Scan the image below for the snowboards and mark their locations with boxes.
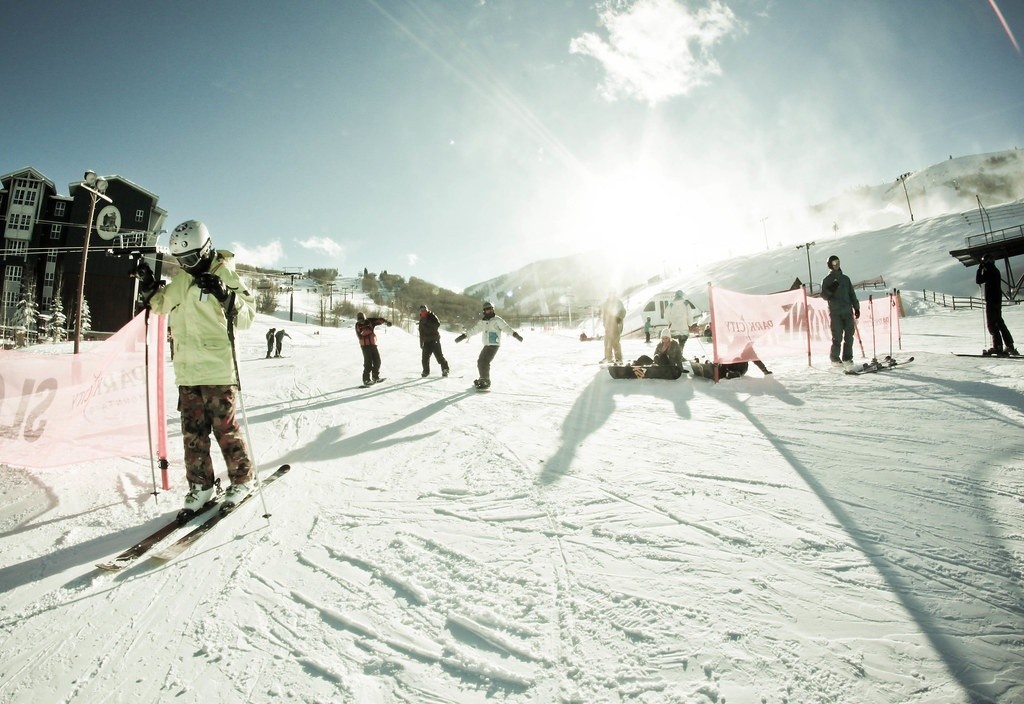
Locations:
[274,356,292,359]
[404,375,463,379]
[358,377,387,389]
[474,376,492,393]
[259,355,276,359]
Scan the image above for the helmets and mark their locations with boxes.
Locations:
[982,254,995,267]
[483,302,494,310]
[827,256,838,268]
[168,220,212,259]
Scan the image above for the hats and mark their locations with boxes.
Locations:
[661,329,670,337]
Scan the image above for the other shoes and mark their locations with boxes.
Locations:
[184,483,214,512]
[373,377,382,382]
[442,369,449,376]
[365,380,373,385]
[223,480,253,503]
[474,380,489,389]
[989,347,1003,353]
[422,373,428,377]
[1004,346,1013,353]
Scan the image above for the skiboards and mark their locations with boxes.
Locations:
[96,463,290,581]
[842,357,915,375]
[950,351,1024,358]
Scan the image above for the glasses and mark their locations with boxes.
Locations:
[832,260,839,266]
[176,253,200,268]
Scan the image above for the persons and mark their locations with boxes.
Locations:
[976,254,1014,354]
[820,256,860,366]
[598,290,772,379]
[266,328,291,358]
[418,305,450,377]
[130,219,256,522]
[355,312,393,385]
[454,301,523,389]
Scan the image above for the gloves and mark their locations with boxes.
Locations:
[831,281,840,290]
[455,334,465,343]
[196,272,228,302]
[141,270,157,293]
[512,332,523,342]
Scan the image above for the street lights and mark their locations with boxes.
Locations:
[895,171,920,224]
[72,169,113,353]
[796,240,819,295]
